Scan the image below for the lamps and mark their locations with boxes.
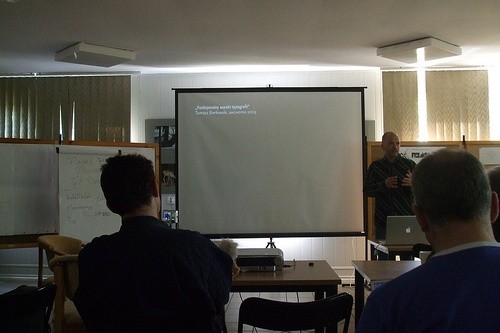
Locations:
[374,37,464,67]
[53,42,135,69]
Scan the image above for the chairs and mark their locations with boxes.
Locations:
[238,292,353,333]
[35,234,85,333]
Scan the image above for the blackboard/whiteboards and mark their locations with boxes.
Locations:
[0,137,60,249]
[59,140,160,244]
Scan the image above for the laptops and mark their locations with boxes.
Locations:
[385,215,427,246]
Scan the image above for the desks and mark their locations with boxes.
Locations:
[351,259,424,333]
[226,259,342,333]
[365,237,431,291]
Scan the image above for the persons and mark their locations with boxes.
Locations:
[363,131,417,241]
[73,152,231,333]
[359,147,499,333]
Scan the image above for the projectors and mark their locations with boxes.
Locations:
[236,248,285,272]
